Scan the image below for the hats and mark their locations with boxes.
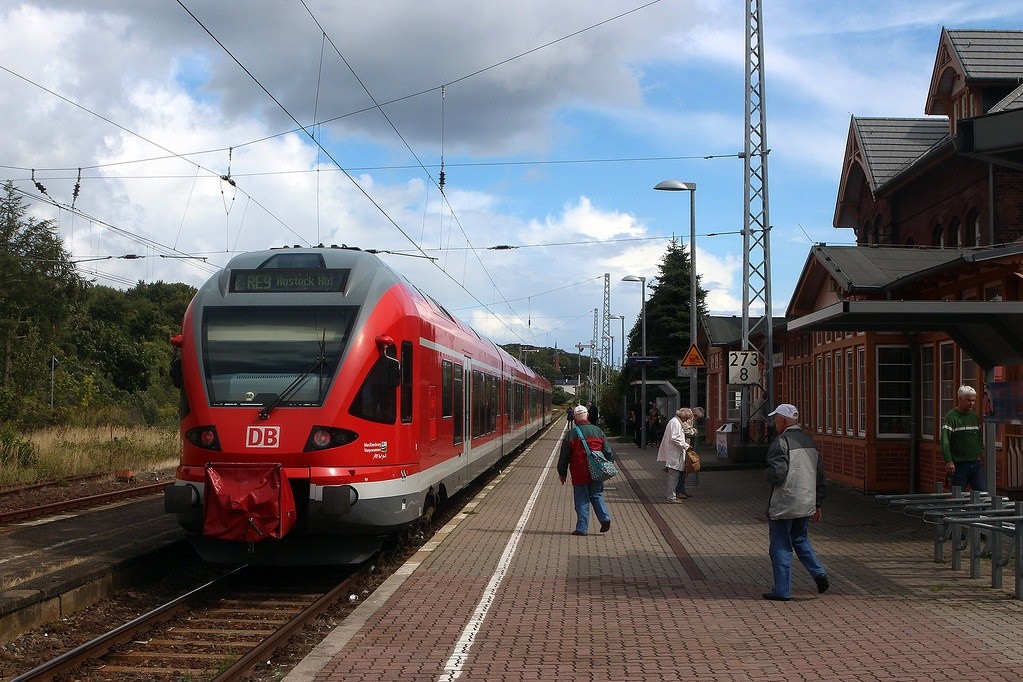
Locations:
[574,405,588,415]
[768,403,798,419]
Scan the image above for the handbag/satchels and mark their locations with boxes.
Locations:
[587,450,618,482]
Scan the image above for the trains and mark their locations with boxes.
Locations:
[163,242,554,559]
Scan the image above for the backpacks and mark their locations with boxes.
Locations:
[685,449,700,473]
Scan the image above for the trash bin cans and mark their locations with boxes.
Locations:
[716,423,740,460]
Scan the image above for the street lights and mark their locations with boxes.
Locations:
[621,275,647,448]
[653,179,699,486]
[595,313,628,439]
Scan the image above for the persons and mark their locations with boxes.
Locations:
[940,385,987,540]
[658,406,705,504]
[567,404,574,430]
[588,403,599,426]
[557,405,614,535]
[631,398,661,447]
[761,403,831,601]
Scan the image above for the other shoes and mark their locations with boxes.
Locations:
[676,491,688,499]
[668,496,683,504]
[761,591,790,601]
[682,490,692,497]
[817,573,829,594]
[600,520,610,532]
[980,532,988,541]
[572,530,587,536]
[949,531,966,541]
